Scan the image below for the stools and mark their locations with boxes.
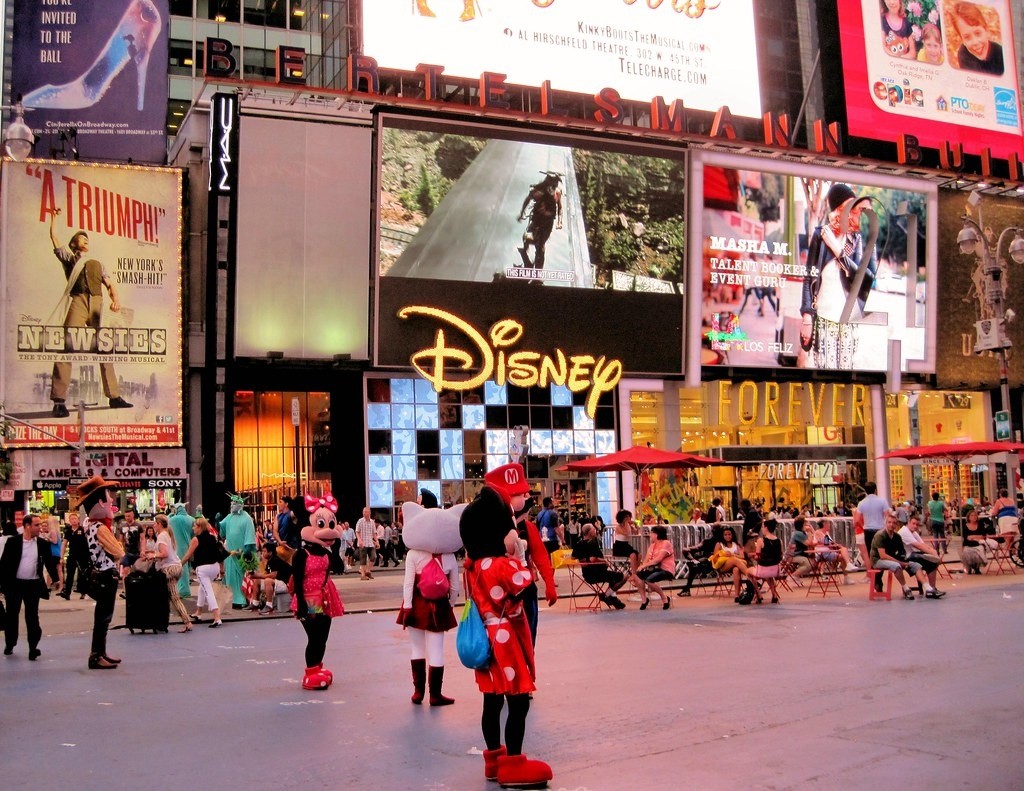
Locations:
[275,592,292,612]
[697,560,802,601]
[584,555,674,611]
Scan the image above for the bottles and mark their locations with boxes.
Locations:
[824,535,829,545]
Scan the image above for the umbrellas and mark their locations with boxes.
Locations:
[555,445,725,524]
[876,441,1024,536]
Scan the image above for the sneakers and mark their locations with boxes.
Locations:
[677,587,691,597]
[901,586,946,600]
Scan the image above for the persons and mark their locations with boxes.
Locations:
[881,0,1004,76]
[852,482,949,600]
[528,497,676,611]
[113,491,299,633]
[795,184,876,370]
[677,517,855,605]
[641,494,852,526]
[517,170,563,269]
[961,509,989,575]
[286,496,343,690]
[737,185,780,317]
[0,511,91,662]
[49,208,133,419]
[945,489,1024,552]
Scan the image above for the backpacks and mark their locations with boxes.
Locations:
[78,563,106,603]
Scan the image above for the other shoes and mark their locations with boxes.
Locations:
[47,581,85,600]
[843,575,870,585]
[119,591,126,599]
[640,596,670,610]
[242,602,275,613]
[734,595,778,604]
[177,613,223,633]
[968,568,982,574]
[786,574,804,584]
[347,560,402,580]
[4,644,41,661]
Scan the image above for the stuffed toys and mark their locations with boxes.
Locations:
[459,463,558,786]
[885,30,909,57]
[393,488,470,707]
[70,475,125,670]
[329,502,465,581]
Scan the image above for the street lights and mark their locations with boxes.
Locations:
[957,214,1024,441]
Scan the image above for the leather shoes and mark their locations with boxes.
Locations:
[89,654,121,669]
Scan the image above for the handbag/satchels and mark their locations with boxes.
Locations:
[0,600,7,631]
[217,541,231,563]
[550,544,580,569]
[133,557,152,572]
[922,552,942,564]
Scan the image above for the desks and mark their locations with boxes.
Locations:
[566,562,607,612]
[985,533,1018,575]
[923,538,953,581]
[805,549,844,597]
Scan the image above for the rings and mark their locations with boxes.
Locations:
[803,337,807,341]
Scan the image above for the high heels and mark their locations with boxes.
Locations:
[599,592,626,609]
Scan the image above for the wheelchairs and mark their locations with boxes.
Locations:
[1010,535,1024,567]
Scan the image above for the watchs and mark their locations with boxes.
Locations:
[153,554,157,559]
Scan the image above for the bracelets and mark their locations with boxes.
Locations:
[60,556,64,559]
[802,321,813,325]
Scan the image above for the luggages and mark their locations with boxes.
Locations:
[124,570,170,634]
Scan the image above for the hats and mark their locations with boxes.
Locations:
[75,475,120,508]
